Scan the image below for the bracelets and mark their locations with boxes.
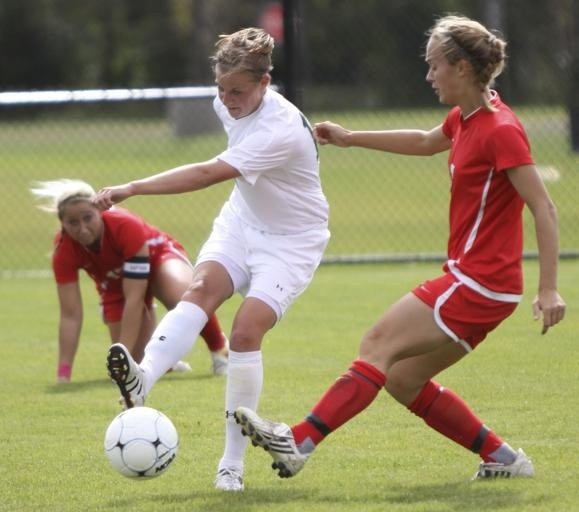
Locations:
[57,361,73,380]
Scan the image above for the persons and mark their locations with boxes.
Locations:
[90,25,327,492]
[232,11,568,485]
[29,176,230,388]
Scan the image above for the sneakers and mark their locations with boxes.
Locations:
[212,464,247,498]
[210,331,232,380]
[171,358,196,374]
[233,404,315,481]
[105,341,148,413]
[467,445,537,484]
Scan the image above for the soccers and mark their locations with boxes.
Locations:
[105,408,179,481]
